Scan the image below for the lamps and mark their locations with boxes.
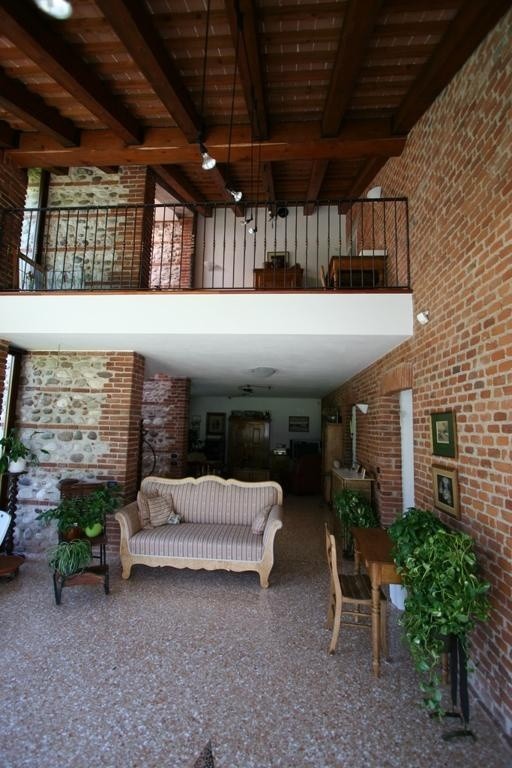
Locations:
[197,27,263,235]
[366,185,382,202]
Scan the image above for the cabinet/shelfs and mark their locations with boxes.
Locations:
[52,479,110,605]
[252,268,304,288]
[321,421,344,473]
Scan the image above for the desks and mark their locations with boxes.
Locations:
[350,526,408,678]
[328,466,376,511]
[325,255,388,290]
[1,471,27,579]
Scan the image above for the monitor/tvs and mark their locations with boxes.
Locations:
[292,440,321,460]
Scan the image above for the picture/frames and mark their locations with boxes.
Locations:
[206,412,226,436]
[428,409,463,522]
[267,250,289,265]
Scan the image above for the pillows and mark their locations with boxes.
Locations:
[137,488,174,529]
[250,504,273,536]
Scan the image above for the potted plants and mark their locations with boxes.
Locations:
[0,426,51,474]
[35,482,122,578]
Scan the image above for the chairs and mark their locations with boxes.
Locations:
[323,521,393,664]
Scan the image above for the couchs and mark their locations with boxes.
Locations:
[114,475,285,589]
